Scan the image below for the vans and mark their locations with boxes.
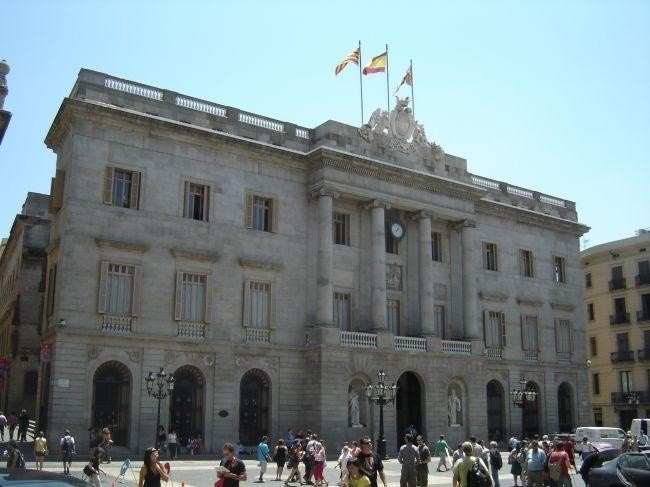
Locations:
[575,425,641,457]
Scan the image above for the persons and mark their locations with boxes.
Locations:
[434,429,502,487]
[508,430,649,486]
[393,65,412,94]
[1,410,111,486]
[363,51,387,75]
[139,425,247,487]
[335,47,360,75]
[258,428,387,486]
[398,422,431,486]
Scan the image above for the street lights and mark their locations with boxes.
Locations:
[361,368,400,460]
[509,375,538,448]
[628,392,639,425]
[144,366,177,460]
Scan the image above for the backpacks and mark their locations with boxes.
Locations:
[516,453,525,463]
[16,451,27,473]
[467,460,492,487]
[492,450,502,470]
[63,436,74,452]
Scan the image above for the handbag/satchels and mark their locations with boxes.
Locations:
[83,465,92,476]
[265,455,272,462]
[44,450,49,457]
[215,475,225,487]
[508,454,515,464]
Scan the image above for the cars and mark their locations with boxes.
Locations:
[588,450,650,487]
[552,432,576,451]
[0,471,96,486]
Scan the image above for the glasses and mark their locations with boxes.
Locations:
[362,441,373,444]
[151,449,157,453]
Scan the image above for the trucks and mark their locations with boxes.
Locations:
[631,417,650,445]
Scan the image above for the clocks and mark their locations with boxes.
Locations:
[391,222,404,241]
